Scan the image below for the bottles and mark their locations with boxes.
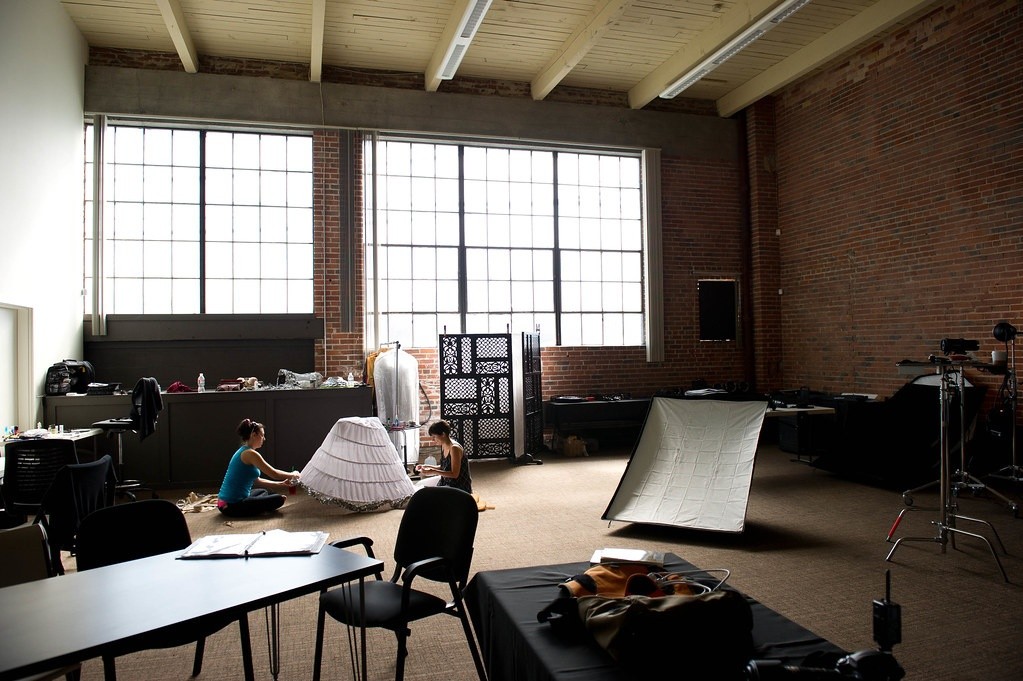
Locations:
[198,373,205,393]
[347,372,354,387]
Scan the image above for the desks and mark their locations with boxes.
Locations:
[464,548,887,681]
[763,400,835,464]
[0,526,383,681]
[1,426,105,466]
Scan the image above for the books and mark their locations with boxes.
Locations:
[600,547,666,567]
[589,549,604,566]
[175,529,331,560]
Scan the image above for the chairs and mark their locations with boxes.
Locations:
[2,437,84,518]
[0,521,81,681]
[35,456,116,553]
[308,485,487,681]
[94,378,162,493]
[73,502,255,681]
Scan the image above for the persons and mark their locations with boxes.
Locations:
[414,420,496,512]
[217,418,301,518]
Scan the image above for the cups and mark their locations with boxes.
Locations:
[991,350,1008,366]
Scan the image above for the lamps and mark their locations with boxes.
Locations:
[991,321,1023,482]
